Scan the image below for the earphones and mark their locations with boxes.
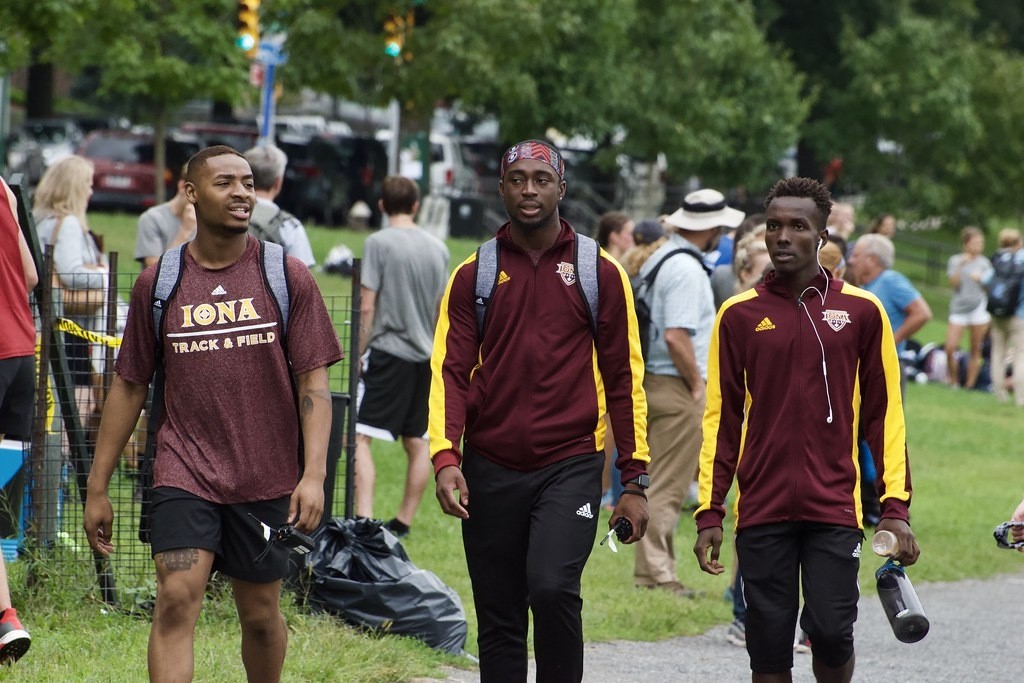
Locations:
[826,409,833,423]
[818,239,823,250]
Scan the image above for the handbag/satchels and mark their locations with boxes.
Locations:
[633,248,713,363]
[49,216,105,314]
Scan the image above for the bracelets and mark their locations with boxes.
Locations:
[619,489,647,501]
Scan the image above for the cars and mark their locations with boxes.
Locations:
[5,105,667,233]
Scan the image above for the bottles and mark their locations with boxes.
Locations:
[875,556,930,643]
[870,529,900,554]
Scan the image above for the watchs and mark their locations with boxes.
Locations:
[625,474,649,488]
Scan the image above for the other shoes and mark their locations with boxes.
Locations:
[653,582,705,600]
[728,620,747,648]
[796,631,813,657]
[124,463,140,480]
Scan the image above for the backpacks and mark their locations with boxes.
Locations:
[987,249,1024,318]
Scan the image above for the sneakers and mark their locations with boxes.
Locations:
[0,607,32,666]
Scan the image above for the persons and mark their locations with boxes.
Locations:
[634,190,744,596]
[431,138,650,683]
[592,187,1024,549]
[349,176,448,540]
[691,177,920,683]
[33,144,315,502]
[82,144,345,683]
[0,176,38,666]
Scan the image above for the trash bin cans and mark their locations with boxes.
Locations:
[319,386,350,524]
[444,191,483,240]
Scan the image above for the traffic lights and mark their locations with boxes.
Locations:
[383,13,403,58]
[234,0,257,52]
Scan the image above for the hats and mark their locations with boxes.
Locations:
[817,243,846,281]
[666,189,746,232]
[633,220,671,246]
